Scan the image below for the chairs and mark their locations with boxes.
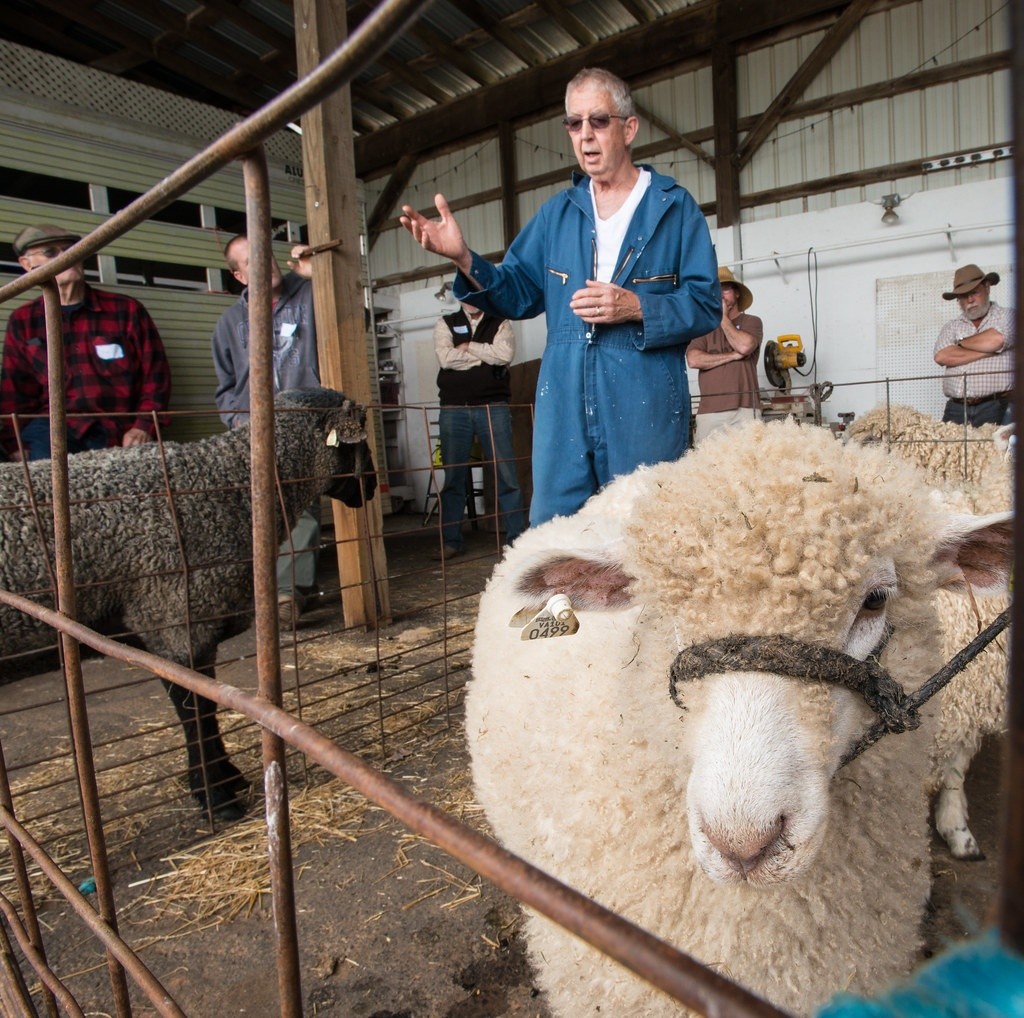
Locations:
[423,421,485,531]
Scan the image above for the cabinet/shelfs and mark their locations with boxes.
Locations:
[372,292,414,503]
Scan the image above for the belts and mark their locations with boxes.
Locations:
[952,391,1015,406]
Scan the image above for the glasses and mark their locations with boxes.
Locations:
[27,245,71,258]
[722,286,736,295]
[561,110,627,132]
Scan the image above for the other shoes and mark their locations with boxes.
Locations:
[433,546,459,562]
[279,597,299,632]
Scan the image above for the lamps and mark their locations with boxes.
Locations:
[880,194,899,225]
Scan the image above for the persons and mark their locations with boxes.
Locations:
[435,301,529,561]
[213,235,323,632]
[400,68,724,532]
[687,266,764,447]
[0,225,172,462]
[933,264,1015,427]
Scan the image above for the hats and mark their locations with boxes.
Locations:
[14,223,81,256]
[943,264,1000,300]
[716,265,753,312]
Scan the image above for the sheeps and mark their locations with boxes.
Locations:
[464,419,1013,1018]
[839,403,1015,520]
[0,387,379,824]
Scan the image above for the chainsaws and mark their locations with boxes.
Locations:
[763,334,807,393]
